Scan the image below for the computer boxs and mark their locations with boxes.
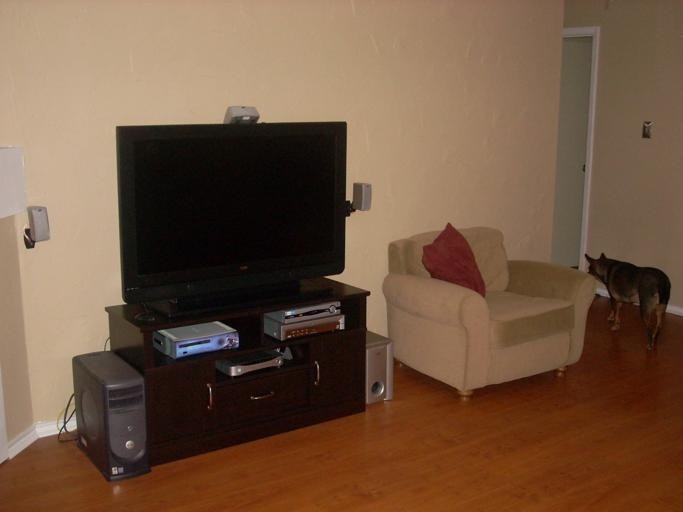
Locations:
[71,351,151,481]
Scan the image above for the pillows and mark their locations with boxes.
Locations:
[421,220,487,300]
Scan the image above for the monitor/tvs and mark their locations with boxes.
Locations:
[115,120,348,318]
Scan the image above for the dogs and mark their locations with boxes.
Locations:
[584,251,671,350]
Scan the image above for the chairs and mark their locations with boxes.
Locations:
[383,225,600,398]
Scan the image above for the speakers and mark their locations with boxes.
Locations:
[26,206,50,243]
[352,182,371,211]
[365,330,394,403]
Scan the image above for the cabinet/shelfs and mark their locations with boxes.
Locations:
[104,277,370,468]
